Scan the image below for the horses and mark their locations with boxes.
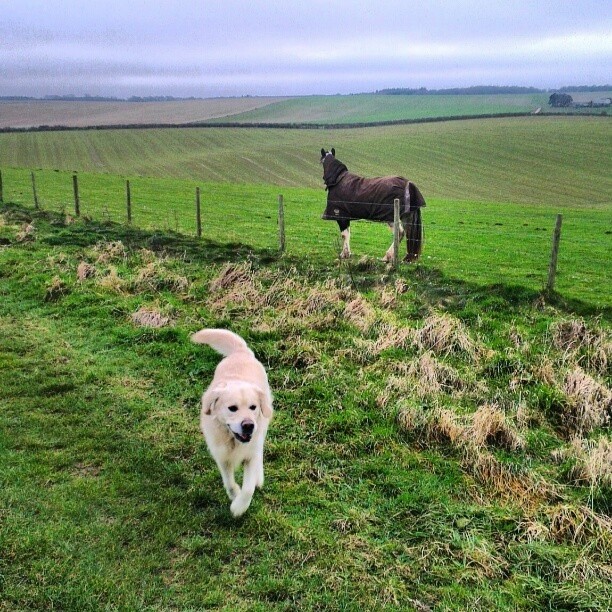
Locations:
[320,148,426,262]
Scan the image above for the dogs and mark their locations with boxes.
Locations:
[190,328,275,517]
